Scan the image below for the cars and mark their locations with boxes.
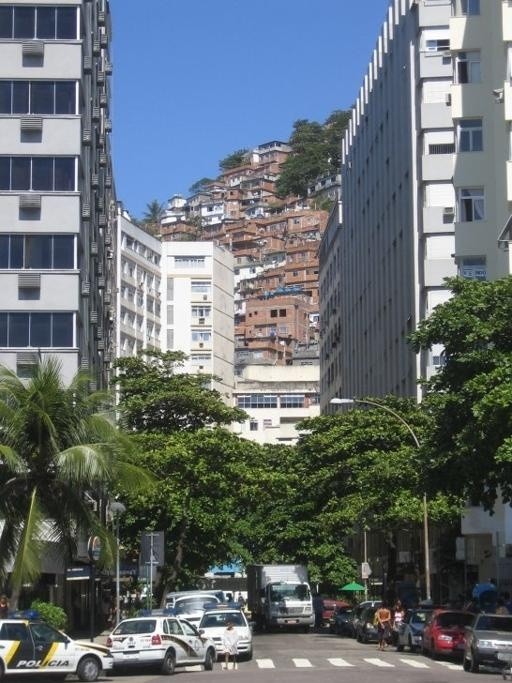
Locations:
[317,598,511,682]
[106,587,255,673]
[0,610,112,683]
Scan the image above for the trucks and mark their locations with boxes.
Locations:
[245,562,317,633]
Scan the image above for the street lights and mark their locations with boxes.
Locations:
[327,397,435,606]
[109,502,126,625]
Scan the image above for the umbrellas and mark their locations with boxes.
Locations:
[339,579,368,591]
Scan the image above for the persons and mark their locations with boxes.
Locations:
[0,595,10,619]
[373,596,406,652]
[467,595,481,614]
[220,618,240,670]
[495,599,511,616]
[314,592,325,632]
